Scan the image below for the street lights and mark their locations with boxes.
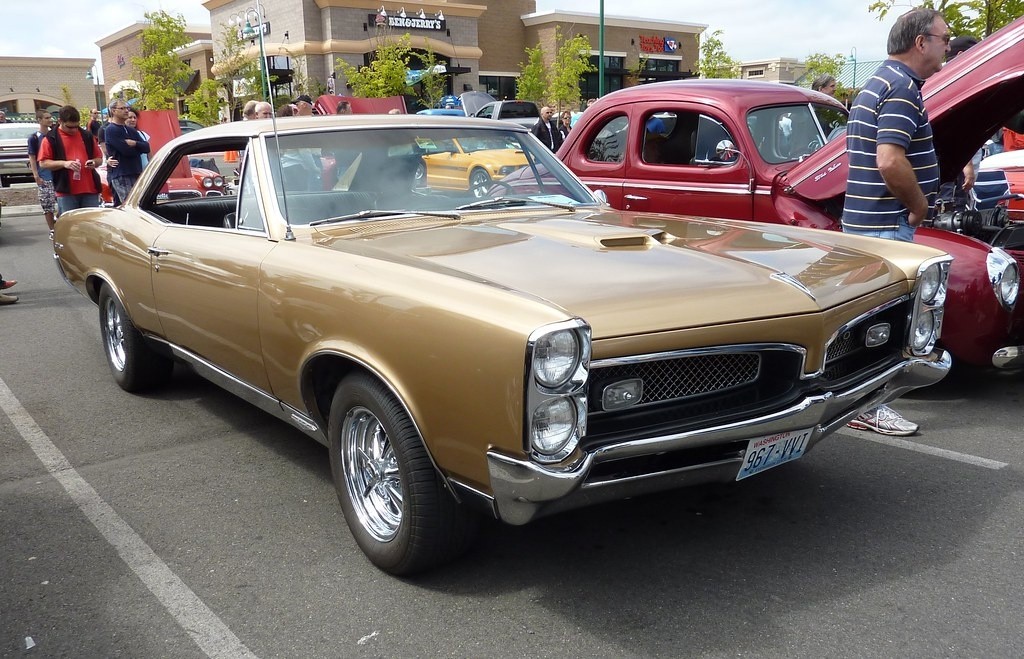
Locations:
[847,46,856,103]
[243,10,267,102]
[86,64,101,111]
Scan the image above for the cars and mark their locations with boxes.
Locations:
[415,108,466,116]
[313,94,410,190]
[415,136,536,199]
[0,121,41,188]
[48,115,952,578]
[96,108,226,207]
[178,120,239,183]
[488,17,1024,392]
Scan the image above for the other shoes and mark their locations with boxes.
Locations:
[1,279,18,290]
[49,229,55,240]
[0,293,19,304]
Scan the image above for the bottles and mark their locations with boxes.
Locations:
[72,159,81,180]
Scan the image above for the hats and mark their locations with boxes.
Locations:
[290,95,312,105]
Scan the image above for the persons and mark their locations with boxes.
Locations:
[930,34,1024,212]
[533,107,560,163]
[842,6,951,437]
[558,110,572,149]
[747,76,836,164]
[645,115,665,133]
[237,94,353,191]
[0,99,150,305]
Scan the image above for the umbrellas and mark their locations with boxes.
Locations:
[435,94,462,109]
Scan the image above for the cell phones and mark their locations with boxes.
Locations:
[87,161,93,165]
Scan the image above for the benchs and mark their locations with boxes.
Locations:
[643,113,702,163]
[150,189,384,228]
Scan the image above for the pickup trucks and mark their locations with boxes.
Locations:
[459,91,560,148]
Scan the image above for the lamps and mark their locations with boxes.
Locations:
[377,5,444,20]
[229,4,266,26]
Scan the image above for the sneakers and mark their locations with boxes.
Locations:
[846,404,921,436]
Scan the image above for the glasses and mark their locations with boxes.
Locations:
[542,111,553,114]
[921,33,951,45]
[113,107,131,111]
[62,124,79,129]
[92,113,98,114]
[563,117,571,120]
[41,116,52,119]
